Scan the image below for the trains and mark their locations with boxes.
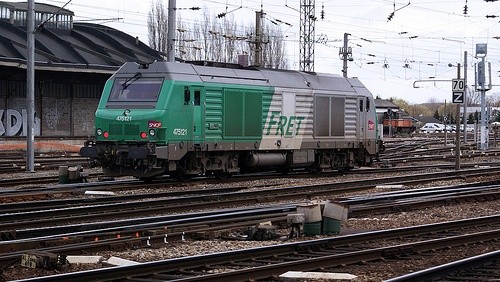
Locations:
[79,60,385,181]
